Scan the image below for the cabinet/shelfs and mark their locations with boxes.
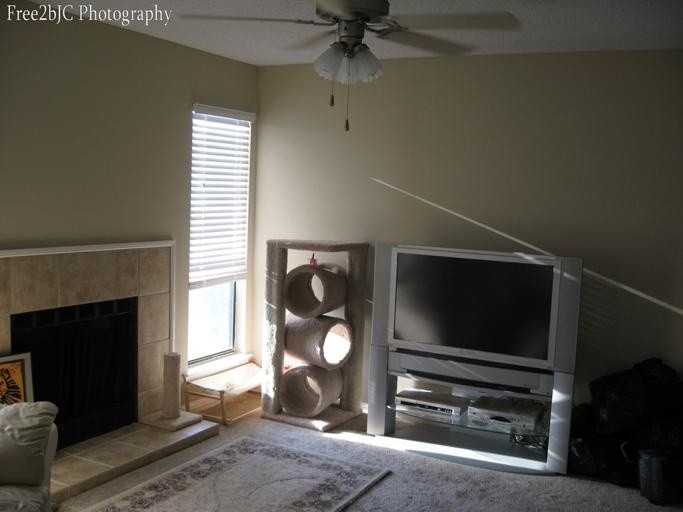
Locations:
[366,343,574,475]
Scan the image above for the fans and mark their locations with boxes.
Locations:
[179,0,522,57]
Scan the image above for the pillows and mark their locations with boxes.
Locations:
[0,401,58,486]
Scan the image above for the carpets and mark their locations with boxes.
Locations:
[79,435,391,512]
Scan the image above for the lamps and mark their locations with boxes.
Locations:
[313,22,383,133]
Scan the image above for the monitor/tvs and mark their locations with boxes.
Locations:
[370,241,584,374]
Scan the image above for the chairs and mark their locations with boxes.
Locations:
[0,403,58,511]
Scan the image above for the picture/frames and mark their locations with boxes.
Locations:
[0,352,34,406]
